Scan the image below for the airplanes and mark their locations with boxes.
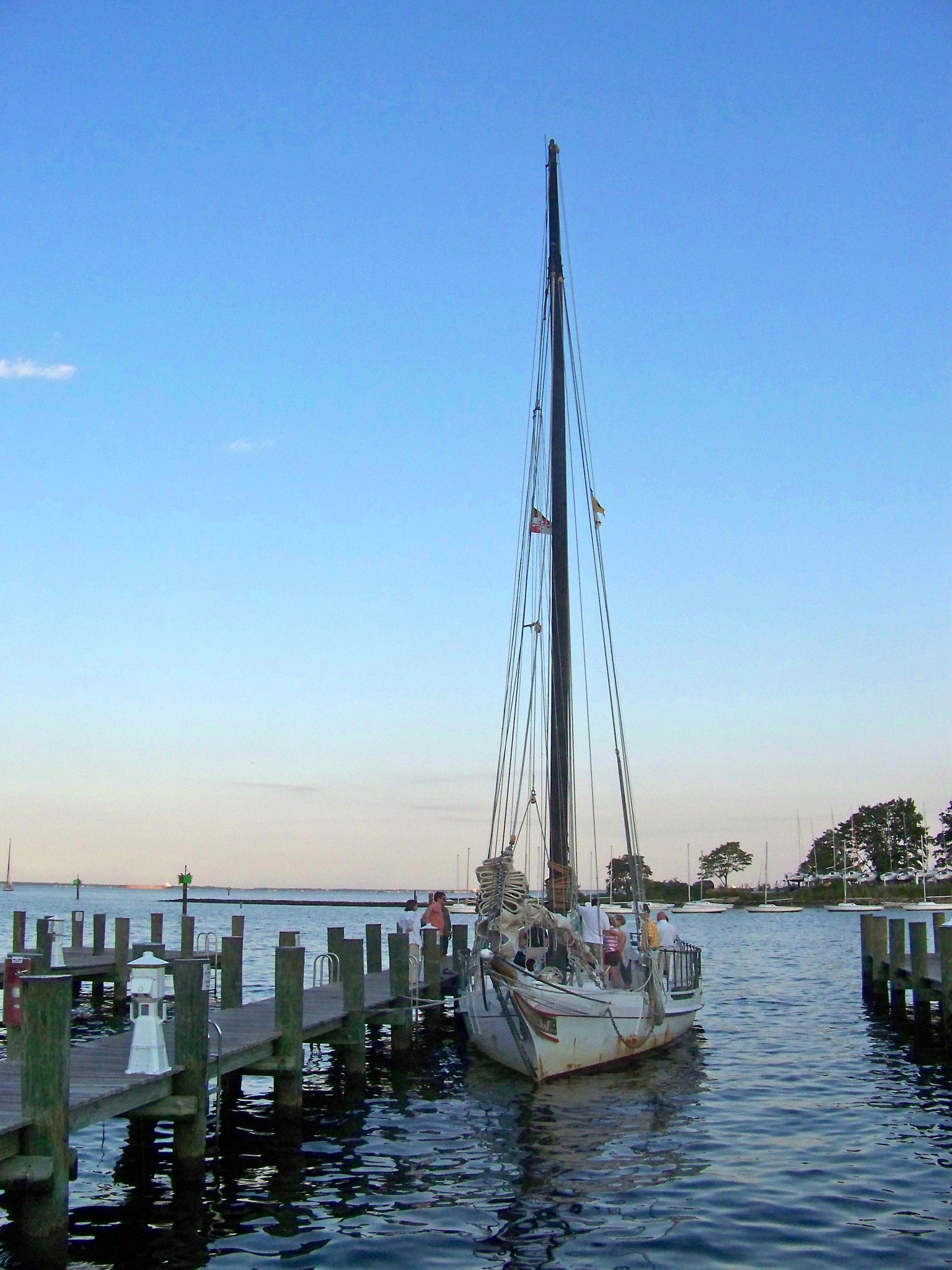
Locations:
[788,866,952,886]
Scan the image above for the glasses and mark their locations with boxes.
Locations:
[441,898,445,900]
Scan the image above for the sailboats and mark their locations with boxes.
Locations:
[444,855,478,913]
[902,834,952,910]
[824,840,885,911]
[585,844,735,914]
[743,842,804,911]
[455,138,709,1075]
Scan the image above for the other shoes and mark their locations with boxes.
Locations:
[443,968,453,974]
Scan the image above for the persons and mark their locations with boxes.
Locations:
[421,892,453,975]
[614,915,637,989]
[639,904,659,950]
[656,911,676,947]
[397,900,421,983]
[576,898,610,964]
[602,916,624,989]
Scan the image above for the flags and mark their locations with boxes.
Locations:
[530,507,552,534]
[592,496,605,516]
